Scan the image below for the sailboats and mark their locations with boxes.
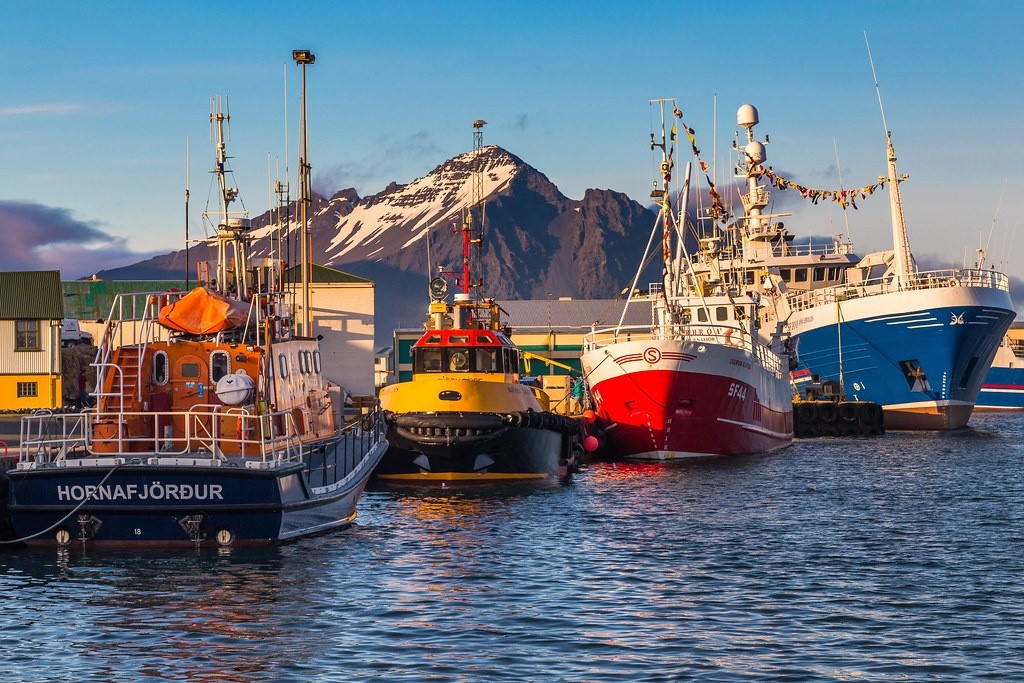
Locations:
[2,35,1022,552]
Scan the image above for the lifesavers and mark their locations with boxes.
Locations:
[725,328,744,348]
[236,412,248,449]
